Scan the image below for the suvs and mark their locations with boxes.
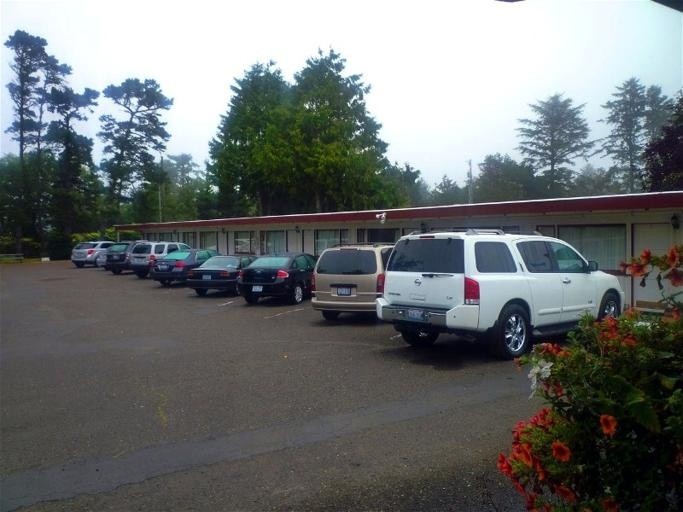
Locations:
[71,240,189,279]
[376,226,626,358]
[311,243,394,323]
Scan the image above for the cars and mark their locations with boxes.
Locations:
[186,256,252,297]
[235,253,319,305]
[147,249,221,288]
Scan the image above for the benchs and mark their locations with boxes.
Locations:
[631,300,669,316]
[0,253,24,264]
